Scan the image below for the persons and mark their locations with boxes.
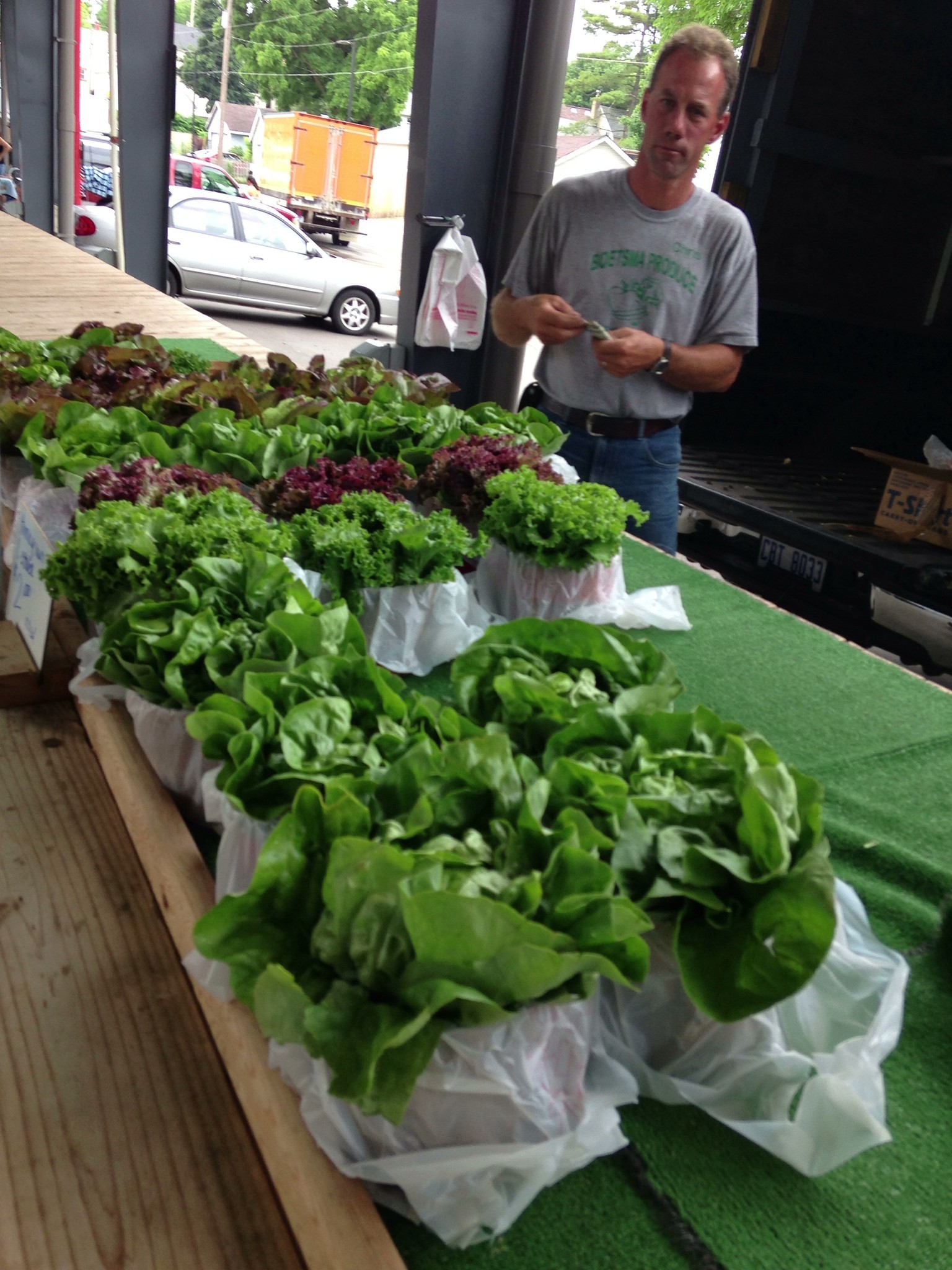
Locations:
[245,173,261,199]
[489,22,759,558]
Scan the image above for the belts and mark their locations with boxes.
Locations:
[538,390,680,440]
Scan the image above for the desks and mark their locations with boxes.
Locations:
[0,211,952,1270]
[675,442,952,674]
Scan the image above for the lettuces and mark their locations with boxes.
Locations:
[35,463,651,638]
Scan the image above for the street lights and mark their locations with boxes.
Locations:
[337,38,357,122]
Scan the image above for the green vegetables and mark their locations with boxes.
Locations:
[0,319,569,529]
[89,541,842,1126]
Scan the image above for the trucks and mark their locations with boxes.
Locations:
[246,111,379,246]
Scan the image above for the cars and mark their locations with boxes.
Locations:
[74,185,401,336]
[80,130,301,231]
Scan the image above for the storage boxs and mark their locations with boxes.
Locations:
[848,446,952,549]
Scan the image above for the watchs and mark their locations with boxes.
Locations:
[649,337,672,377]
